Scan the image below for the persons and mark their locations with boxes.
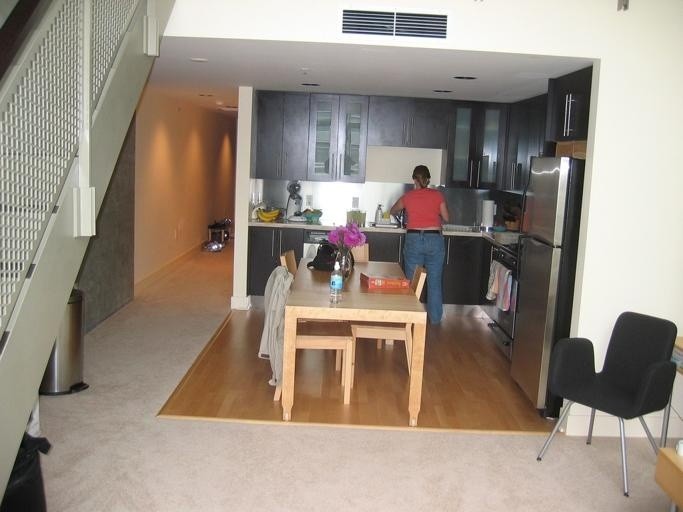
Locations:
[389,166,449,325]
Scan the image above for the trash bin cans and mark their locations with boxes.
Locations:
[39,292,88,395]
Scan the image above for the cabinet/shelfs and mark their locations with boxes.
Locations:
[367,95,450,152]
[246,227,483,306]
[440,99,509,190]
[306,92,369,186]
[253,89,309,182]
[505,65,592,193]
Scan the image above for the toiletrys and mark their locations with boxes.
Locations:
[375,204,384,224]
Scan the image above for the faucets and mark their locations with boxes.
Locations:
[394,209,404,228]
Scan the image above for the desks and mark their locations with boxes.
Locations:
[280,257,427,426]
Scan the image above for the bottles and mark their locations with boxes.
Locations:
[329,260,343,304]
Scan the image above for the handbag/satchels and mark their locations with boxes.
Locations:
[306,240,354,270]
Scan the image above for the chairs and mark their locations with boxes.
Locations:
[536,310,678,497]
[271,241,427,401]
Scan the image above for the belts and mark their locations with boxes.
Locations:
[407,230,440,233]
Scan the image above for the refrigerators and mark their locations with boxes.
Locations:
[508,153,586,421]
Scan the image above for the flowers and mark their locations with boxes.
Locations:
[327,223,366,279]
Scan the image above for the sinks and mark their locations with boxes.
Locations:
[372,223,399,229]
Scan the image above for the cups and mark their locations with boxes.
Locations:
[346,208,366,228]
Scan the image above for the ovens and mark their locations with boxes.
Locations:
[486,245,521,342]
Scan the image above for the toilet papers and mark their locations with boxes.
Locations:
[483,200,494,227]
